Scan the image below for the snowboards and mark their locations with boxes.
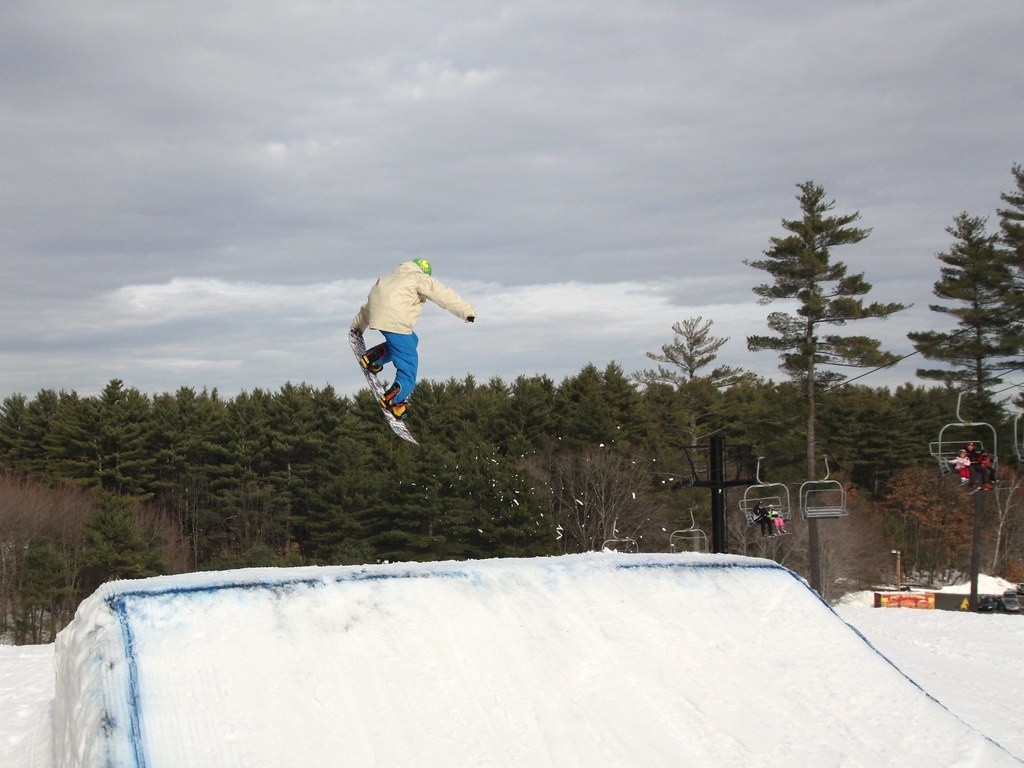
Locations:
[348,328,421,447]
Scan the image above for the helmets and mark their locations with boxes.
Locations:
[412,258,432,277]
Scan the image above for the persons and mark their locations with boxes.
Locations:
[945,441,1001,489]
[350,257,477,421]
[752,499,788,537]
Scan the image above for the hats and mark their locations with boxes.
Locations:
[961,443,989,459]
[757,500,774,509]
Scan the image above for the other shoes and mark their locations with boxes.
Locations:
[959,477,1002,491]
[364,350,383,374]
[760,529,790,538]
[382,393,406,420]
